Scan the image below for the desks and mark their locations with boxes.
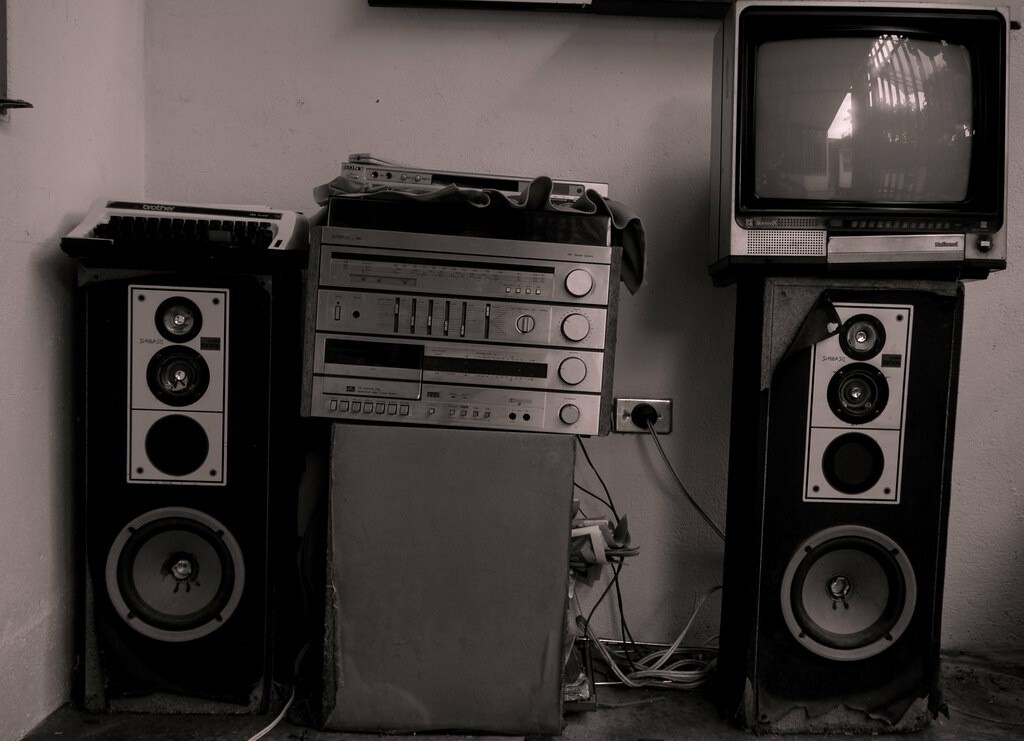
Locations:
[324,422,577,736]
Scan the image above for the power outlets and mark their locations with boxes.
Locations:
[583,518,609,550]
[613,397,673,435]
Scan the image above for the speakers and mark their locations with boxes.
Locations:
[719,278,966,736]
[72,250,327,715]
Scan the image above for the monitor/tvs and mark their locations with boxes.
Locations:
[704,0,1008,279]
